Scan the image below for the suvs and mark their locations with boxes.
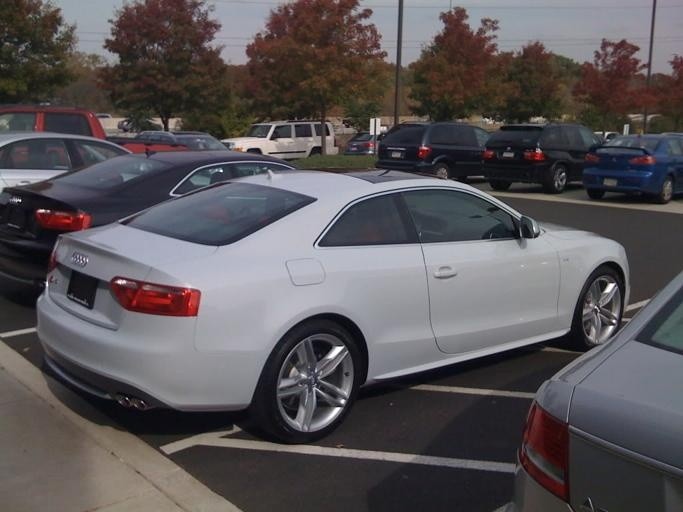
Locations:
[376,122,497,183]
[221,120,338,160]
[482,122,607,193]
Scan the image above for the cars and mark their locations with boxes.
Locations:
[511,269,681,512]
[1,107,227,191]
[0,149,293,307]
[345,131,381,156]
[32,166,630,445]
[582,128,682,204]
